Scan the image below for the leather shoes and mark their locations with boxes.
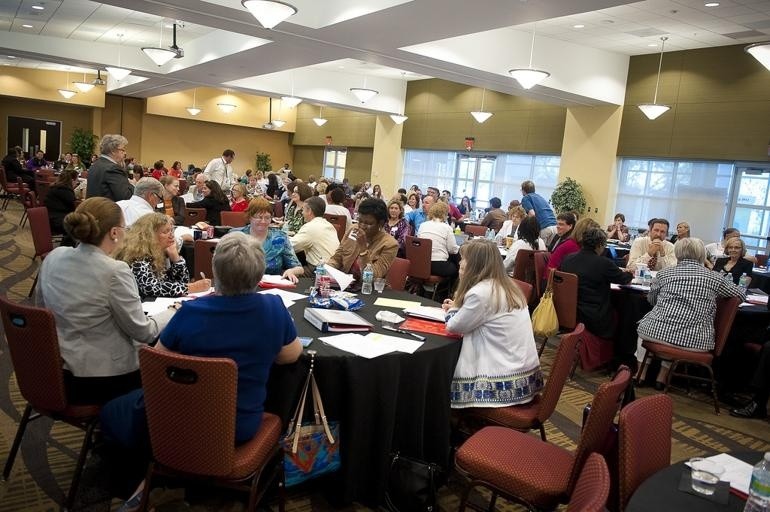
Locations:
[632,376,646,387]
[729,401,766,419]
[655,380,667,391]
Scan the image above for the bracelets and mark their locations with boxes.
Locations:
[617,229,621,232]
[360,249,369,258]
[166,305,180,312]
[660,251,665,257]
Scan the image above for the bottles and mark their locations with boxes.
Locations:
[643,268,650,288]
[489,229,495,239]
[739,272,747,288]
[727,272,733,282]
[314,256,324,289]
[485,227,490,237]
[361,263,372,295]
[744,452,769,512]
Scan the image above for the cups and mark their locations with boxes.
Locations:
[320,288,335,308]
[689,457,726,496]
[373,277,386,294]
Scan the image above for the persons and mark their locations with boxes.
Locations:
[43,170,87,235]
[273,182,299,228]
[712,237,753,291]
[116,213,213,299]
[115,177,164,226]
[384,199,413,261]
[179,174,211,208]
[125,156,203,176]
[546,213,576,252]
[648,217,658,225]
[1,148,36,196]
[404,195,419,213]
[406,196,435,239]
[391,188,407,205]
[521,180,558,246]
[16,143,99,170]
[627,219,677,272]
[543,217,600,291]
[457,196,473,214]
[632,237,746,392]
[704,228,758,271]
[728,342,770,418]
[480,197,507,233]
[204,149,235,195]
[231,185,249,212]
[494,206,526,248]
[416,203,460,303]
[154,175,186,231]
[503,216,548,276]
[436,196,463,224]
[235,163,392,196]
[228,197,304,285]
[560,228,635,366]
[670,222,691,245]
[441,239,544,409]
[325,198,400,290]
[186,180,232,226]
[508,199,520,220]
[281,183,311,237]
[606,214,631,241]
[100,231,304,447]
[289,196,341,278]
[407,185,458,201]
[34,197,183,402]
[85,134,137,202]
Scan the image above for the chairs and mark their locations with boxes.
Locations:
[636,296,740,416]
[192,240,218,281]
[465,225,489,237]
[183,208,206,226]
[471,323,585,442]
[220,211,250,227]
[754,254,769,270]
[389,257,410,291]
[512,249,546,305]
[534,252,550,302]
[405,235,450,301]
[567,451,610,511]
[510,278,533,305]
[322,214,347,243]
[137,347,285,511]
[0,295,106,511]
[454,363,631,511]
[532,267,581,382]
[26,206,67,297]
[618,393,672,512]
[0,166,34,229]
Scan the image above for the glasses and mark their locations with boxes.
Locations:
[155,192,164,201]
[727,245,741,250]
[117,147,127,153]
[117,223,131,232]
[252,214,272,222]
[355,217,374,227]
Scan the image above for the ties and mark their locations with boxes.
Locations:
[649,251,657,270]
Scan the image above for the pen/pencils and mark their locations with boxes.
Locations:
[406,331,426,341]
[199,271,206,279]
[381,325,406,334]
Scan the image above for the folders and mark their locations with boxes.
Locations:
[303,307,375,333]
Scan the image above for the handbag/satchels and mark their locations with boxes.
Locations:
[531,290,559,337]
[383,448,438,510]
[278,420,341,488]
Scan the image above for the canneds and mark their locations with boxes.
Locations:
[320,277,331,298]
[207,226,214,239]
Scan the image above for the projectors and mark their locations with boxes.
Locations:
[92,79,104,87]
[262,123,274,131]
[168,46,185,58]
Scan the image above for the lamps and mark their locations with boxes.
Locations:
[240,0,298,30]
[508,24,550,91]
[271,98,287,127]
[468,88,495,124]
[185,88,202,116]
[743,42,770,71]
[312,106,328,127]
[636,37,672,120]
[56,69,78,99]
[72,73,95,93]
[140,17,177,67]
[281,71,302,109]
[349,76,379,103]
[104,34,132,82]
[388,72,408,125]
[216,89,237,113]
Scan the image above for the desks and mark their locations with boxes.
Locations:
[589,268,769,363]
[622,452,770,512]
[140,277,463,490]
[34,169,89,205]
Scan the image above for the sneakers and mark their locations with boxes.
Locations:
[113,480,145,511]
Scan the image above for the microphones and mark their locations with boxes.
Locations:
[125,164,134,179]
